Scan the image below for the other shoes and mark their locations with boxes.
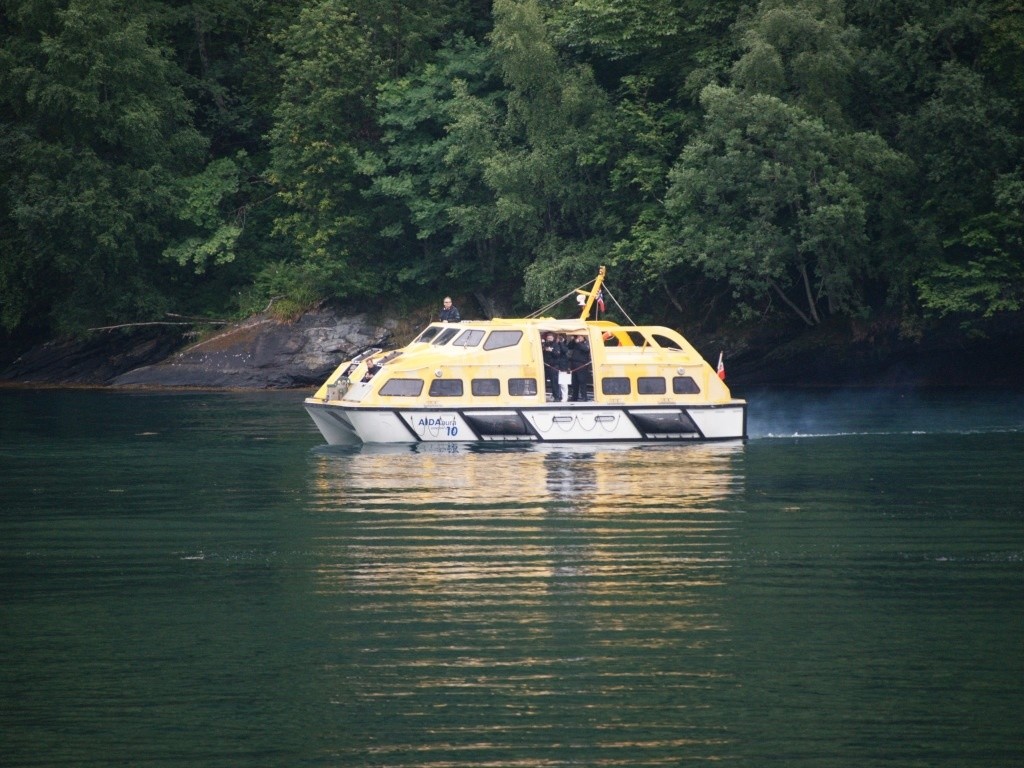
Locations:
[553,398,561,402]
[569,398,578,401]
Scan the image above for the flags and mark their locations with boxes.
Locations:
[718,355,725,380]
[596,289,604,312]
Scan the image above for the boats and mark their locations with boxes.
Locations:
[303,266,748,446]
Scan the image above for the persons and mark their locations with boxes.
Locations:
[364,359,379,380]
[439,296,461,323]
[542,331,590,401]
